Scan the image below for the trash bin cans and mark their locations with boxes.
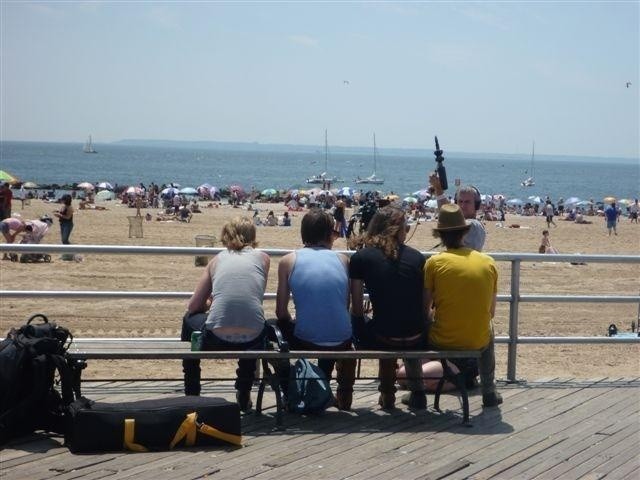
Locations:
[127,215,144,237]
[194,235,216,267]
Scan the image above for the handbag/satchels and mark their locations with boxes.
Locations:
[16,312,74,351]
[65,394,243,454]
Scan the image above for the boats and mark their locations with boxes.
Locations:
[521,176,535,187]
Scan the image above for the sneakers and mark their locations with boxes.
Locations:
[335,392,505,410]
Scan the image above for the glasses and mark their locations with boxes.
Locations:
[406,225,411,233]
[330,227,339,240]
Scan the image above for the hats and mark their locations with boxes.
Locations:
[432,203,473,231]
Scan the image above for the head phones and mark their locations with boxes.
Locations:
[454,185,481,211]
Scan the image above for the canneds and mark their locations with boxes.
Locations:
[190,330,204,352]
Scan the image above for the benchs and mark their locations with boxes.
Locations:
[59,340,479,427]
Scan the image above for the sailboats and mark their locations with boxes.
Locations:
[356,130,386,186]
[306,129,342,184]
[84,130,97,155]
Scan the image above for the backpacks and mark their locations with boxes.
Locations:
[0,335,89,445]
[295,357,334,413]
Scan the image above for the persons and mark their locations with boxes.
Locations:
[53,193,76,245]
[331,205,429,412]
[537,229,552,255]
[180,214,271,413]
[0,217,33,262]
[18,233,32,244]
[428,169,485,251]
[264,210,352,408]
[401,204,503,407]
[395,356,479,394]
[1,169,640,237]
